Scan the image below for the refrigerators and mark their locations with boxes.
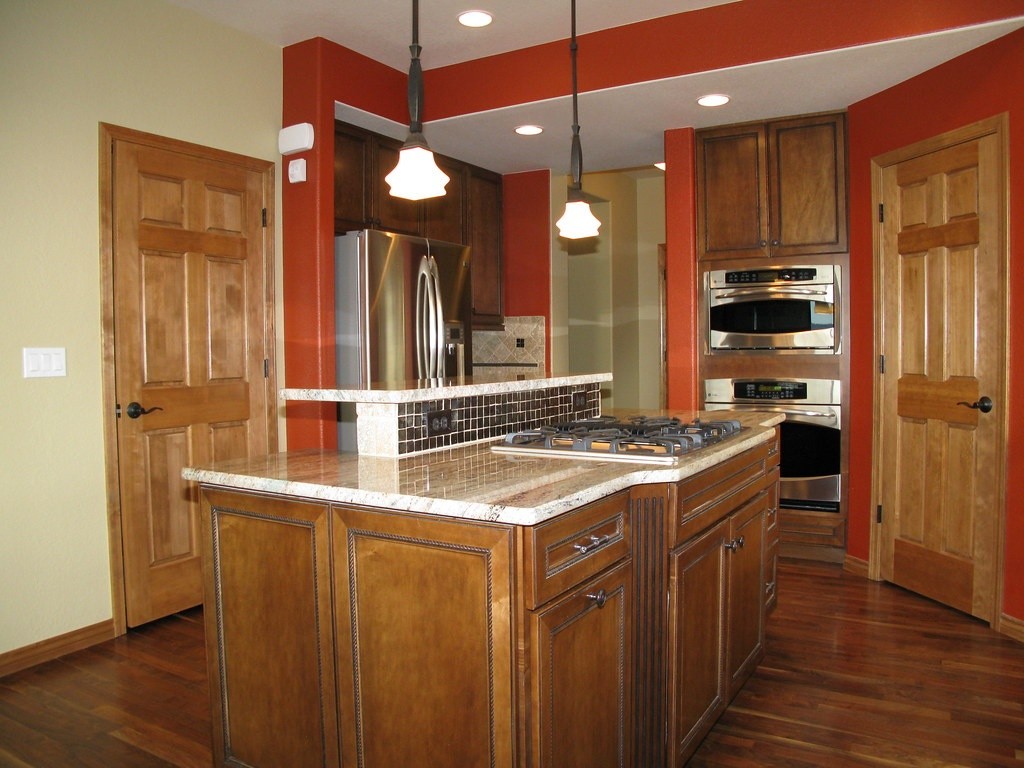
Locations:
[334,228,473,452]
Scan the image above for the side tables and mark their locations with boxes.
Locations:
[181,475,522,768]
[639,407,784,768]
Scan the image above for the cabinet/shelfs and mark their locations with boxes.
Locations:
[333,118,422,236]
[424,147,506,333]
[524,488,635,767]
[694,108,846,260]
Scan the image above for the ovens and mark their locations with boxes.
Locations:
[703,378,843,512]
[702,263,844,357]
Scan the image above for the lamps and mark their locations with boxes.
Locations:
[384,1,451,199]
[554,0,601,240]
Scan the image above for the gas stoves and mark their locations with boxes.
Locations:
[490,413,752,462]
